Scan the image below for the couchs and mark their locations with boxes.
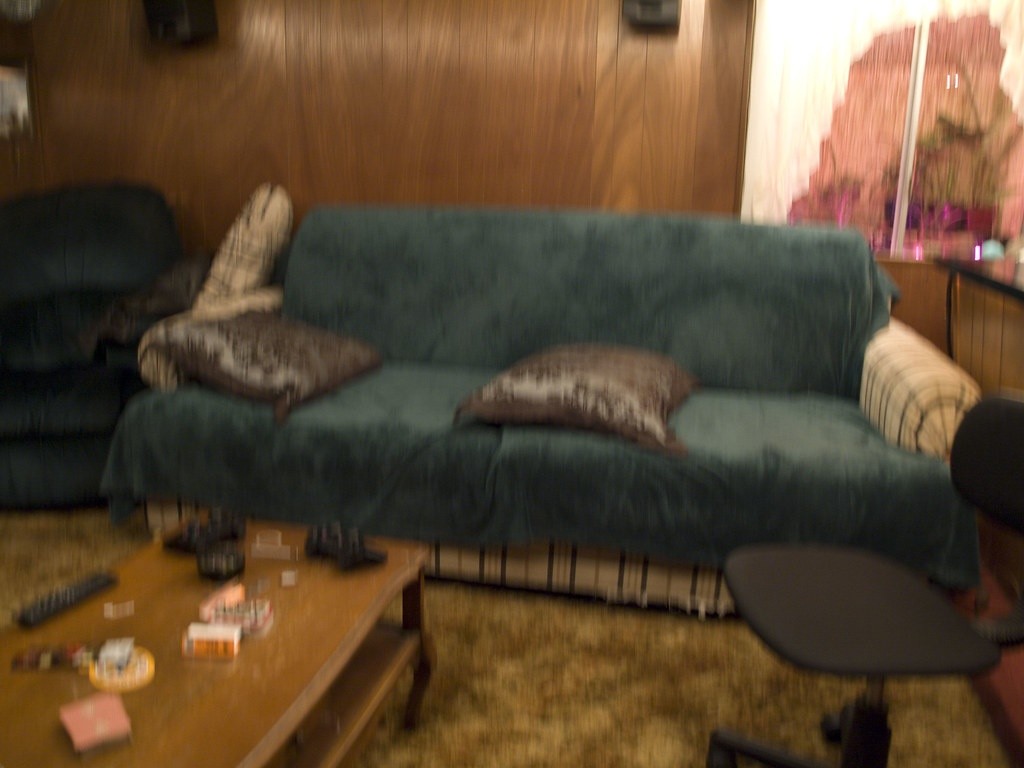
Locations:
[105,204,984,620]
[0,186,204,508]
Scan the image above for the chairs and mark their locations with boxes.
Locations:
[704,397,1024,768]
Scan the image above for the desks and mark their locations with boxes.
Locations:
[935,253,1024,361]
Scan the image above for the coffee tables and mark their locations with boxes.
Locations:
[0,516,435,768]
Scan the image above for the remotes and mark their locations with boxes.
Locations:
[15,572,117,628]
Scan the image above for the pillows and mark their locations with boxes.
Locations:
[167,318,377,403]
[449,342,689,451]
